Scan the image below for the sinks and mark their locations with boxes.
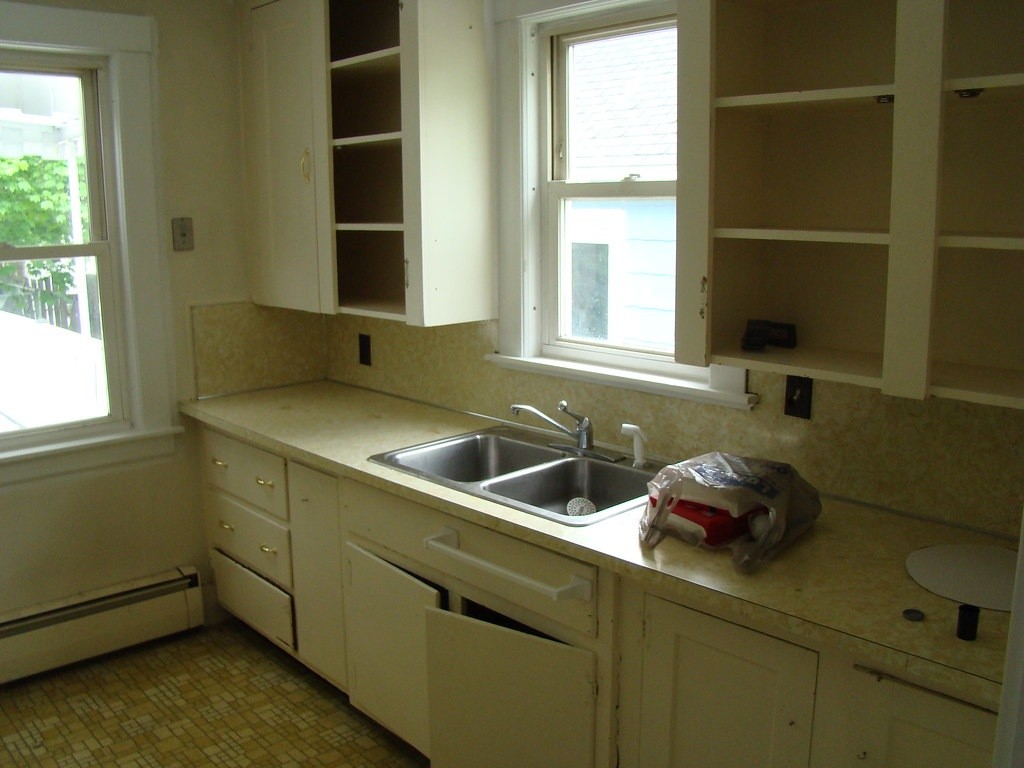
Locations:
[481,458,656,518]
[383,434,568,484]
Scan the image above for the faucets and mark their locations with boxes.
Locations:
[510,399,594,449]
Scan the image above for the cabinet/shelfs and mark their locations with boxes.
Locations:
[674,0,1024,409]
[250,0,499,330]
[195,421,997,768]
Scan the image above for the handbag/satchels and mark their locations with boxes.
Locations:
[639,452,822,570]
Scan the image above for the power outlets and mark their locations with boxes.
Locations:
[172,216,194,250]
